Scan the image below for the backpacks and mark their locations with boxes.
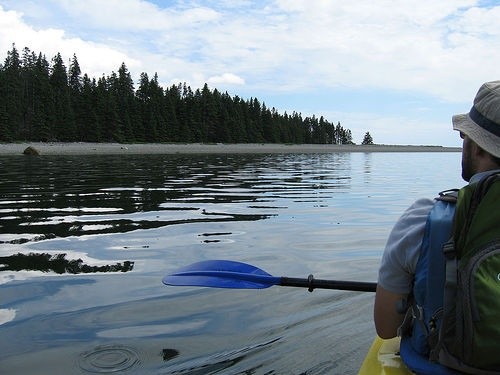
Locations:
[395,169,500,375]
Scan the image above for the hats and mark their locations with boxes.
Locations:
[451,80,500,160]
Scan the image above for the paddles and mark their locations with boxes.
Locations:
[161,260,378,292]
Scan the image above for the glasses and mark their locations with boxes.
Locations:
[459,132,467,139]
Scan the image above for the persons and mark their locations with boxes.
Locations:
[374,81,499,375]
